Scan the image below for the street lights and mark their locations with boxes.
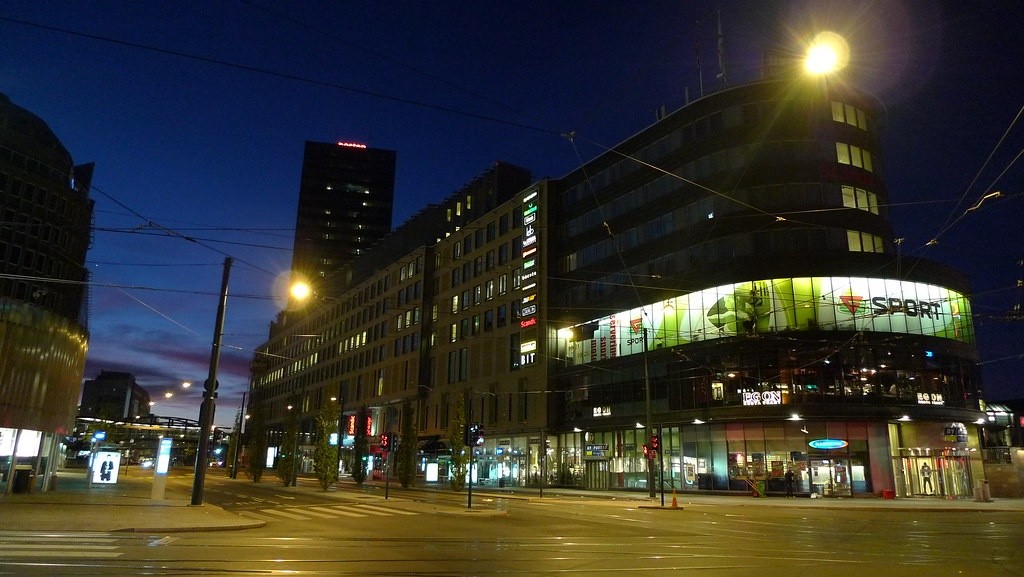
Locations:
[642,304,674,499]
[330,396,344,482]
[231,413,251,478]
[286,404,301,486]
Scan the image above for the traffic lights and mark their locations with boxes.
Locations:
[642,443,648,457]
[651,434,658,451]
[478,423,485,446]
[467,425,475,446]
[381,432,389,448]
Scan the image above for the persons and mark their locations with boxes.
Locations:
[920,462,932,493]
[786,470,795,498]
[100,454,113,481]
